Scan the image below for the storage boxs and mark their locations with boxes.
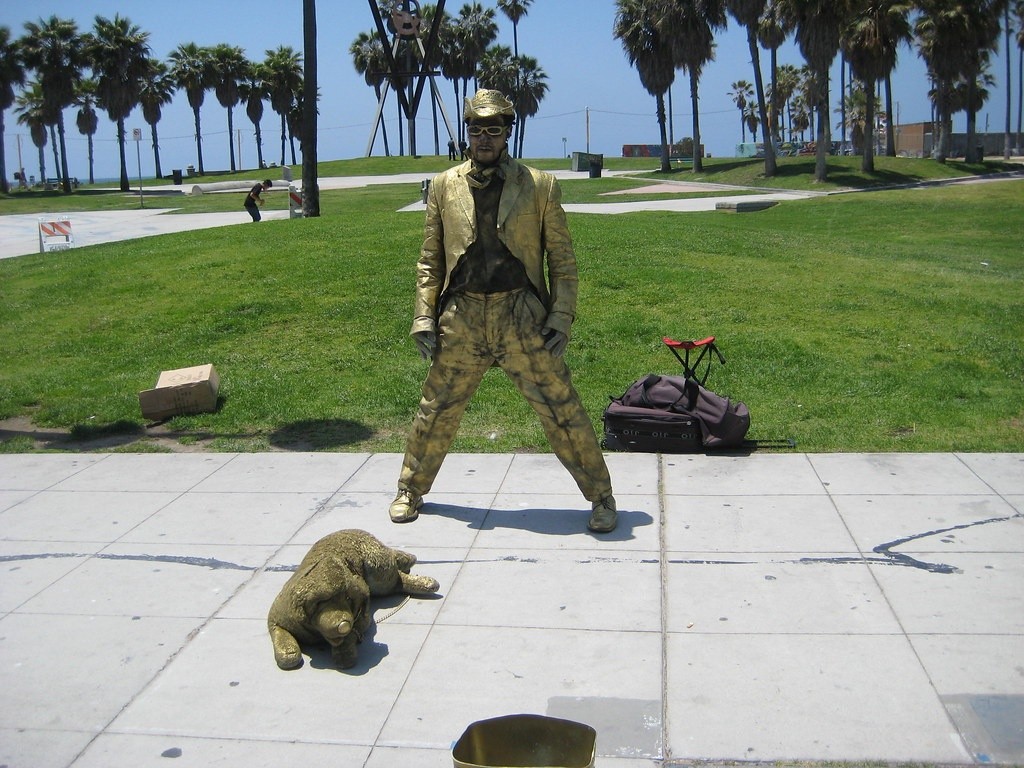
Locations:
[138,364,220,421]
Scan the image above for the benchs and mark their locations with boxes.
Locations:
[47,177,80,190]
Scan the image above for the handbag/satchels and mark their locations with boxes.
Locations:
[609,373,750,448]
[601,400,702,453]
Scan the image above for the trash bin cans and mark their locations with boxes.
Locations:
[589,160,602,177]
[172,169,183,185]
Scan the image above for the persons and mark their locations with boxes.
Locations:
[389,89,618,533]
[19,168,31,191]
[459,139,468,161]
[243,179,273,222]
[448,137,456,161]
[262,160,267,169]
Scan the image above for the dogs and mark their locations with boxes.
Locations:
[266,527,441,671]
